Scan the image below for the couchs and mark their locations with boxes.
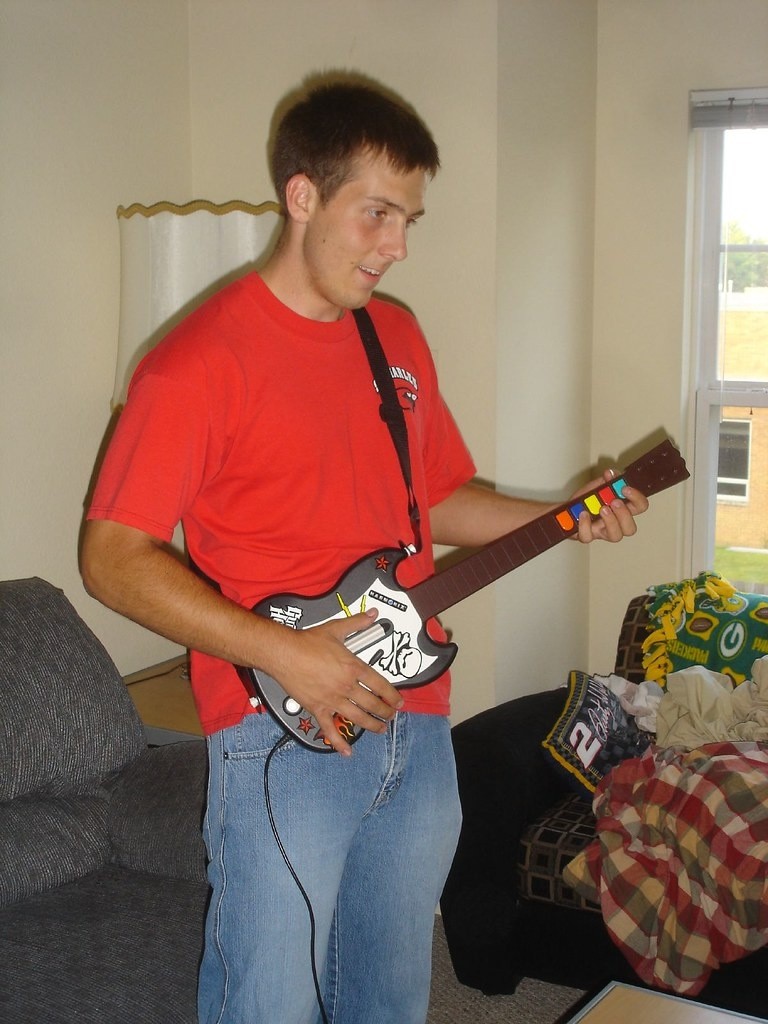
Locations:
[440,572,768,1019]
[0,575,212,1024]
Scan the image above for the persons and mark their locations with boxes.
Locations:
[76,82,649,1024]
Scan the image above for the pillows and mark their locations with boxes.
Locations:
[540,669,653,796]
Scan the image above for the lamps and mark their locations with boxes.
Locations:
[110,200,284,415]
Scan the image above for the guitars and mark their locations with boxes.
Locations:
[248,436,692,756]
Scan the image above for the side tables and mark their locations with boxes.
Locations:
[122,653,207,746]
